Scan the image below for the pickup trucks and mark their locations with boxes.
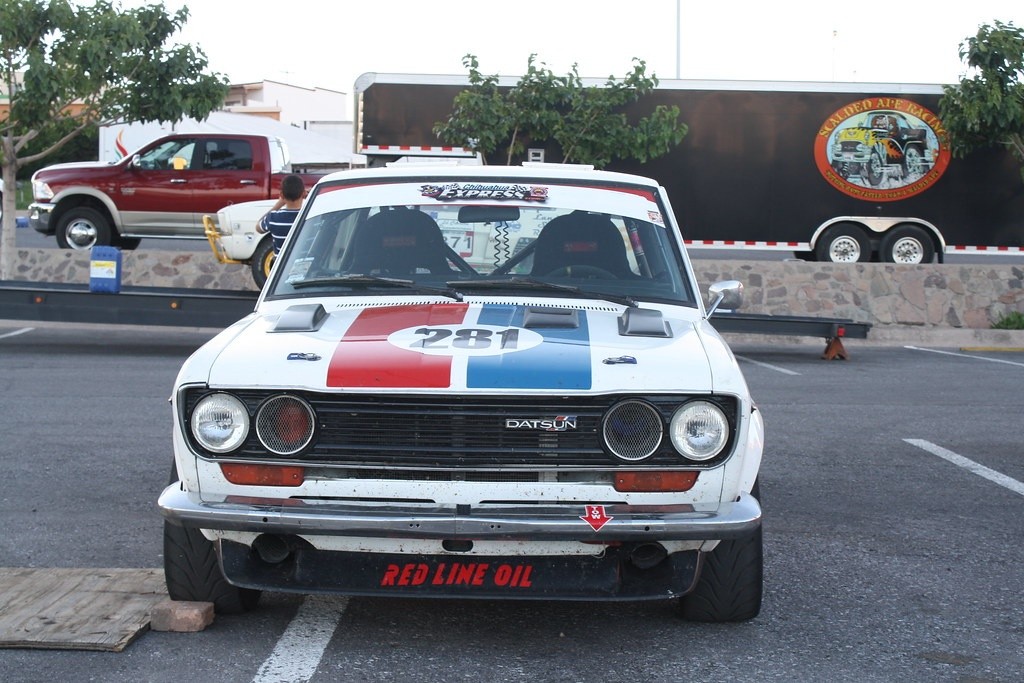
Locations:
[26,130,399,251]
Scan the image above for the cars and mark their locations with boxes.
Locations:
[157,150,768,624]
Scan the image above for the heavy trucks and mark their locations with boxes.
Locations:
[204,70,1024,295]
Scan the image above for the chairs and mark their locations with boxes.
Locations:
[530,213,641,280]
[343,210,452,274]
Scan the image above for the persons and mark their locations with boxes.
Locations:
[256,176,305,263]
